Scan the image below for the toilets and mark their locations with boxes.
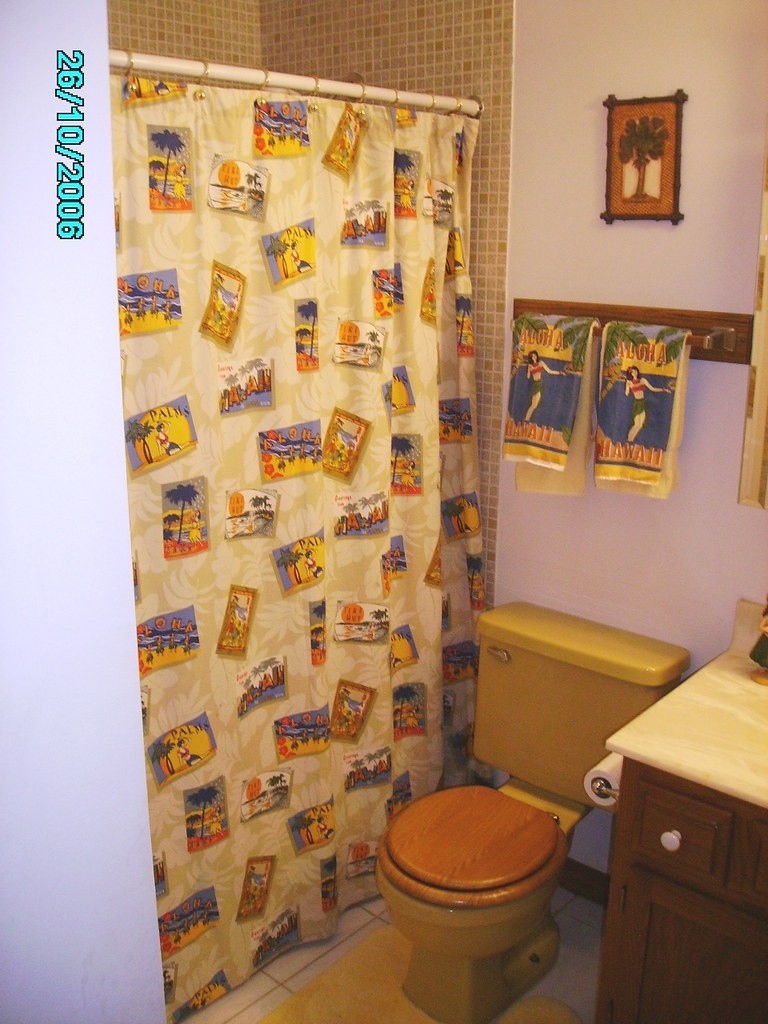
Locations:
[375,600,693,1024]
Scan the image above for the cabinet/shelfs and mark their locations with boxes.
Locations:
[595,755,768,1023]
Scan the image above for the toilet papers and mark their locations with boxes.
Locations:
[583,753,626,809]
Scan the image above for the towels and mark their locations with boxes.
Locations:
[498,313,598,499]
[592,314,694,503]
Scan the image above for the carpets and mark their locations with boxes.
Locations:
[256,923,584,1024]
